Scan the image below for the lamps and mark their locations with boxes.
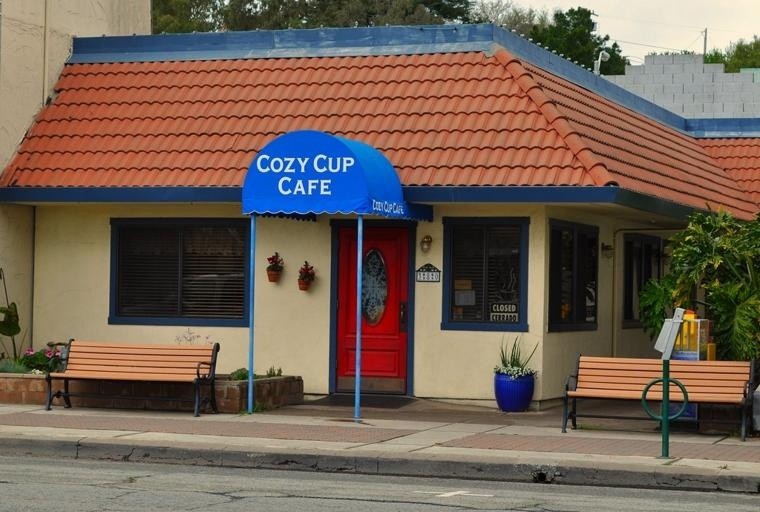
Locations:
[421,235,432,252]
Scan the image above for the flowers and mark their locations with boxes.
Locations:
[491,335,540,381]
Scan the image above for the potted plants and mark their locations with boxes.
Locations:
[265,252,284,283]
[298,260,316,290]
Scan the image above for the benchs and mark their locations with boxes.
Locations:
[559,353,755,442]
[43,338,220,417]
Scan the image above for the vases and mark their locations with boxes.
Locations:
[494,372,534,412]
